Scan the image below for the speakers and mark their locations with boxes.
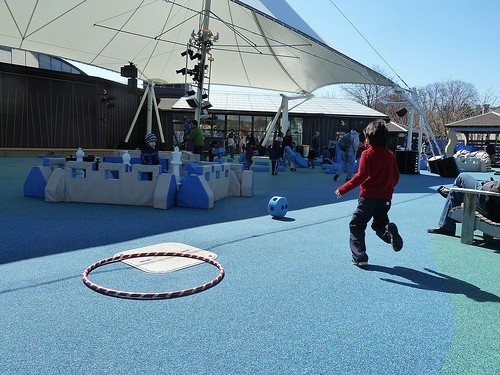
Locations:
[395,107,408,117]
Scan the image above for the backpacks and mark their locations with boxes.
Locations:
[339,132,351,151]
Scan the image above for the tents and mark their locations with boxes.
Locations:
[0,0,443,173]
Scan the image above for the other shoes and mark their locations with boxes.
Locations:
[346,178,350,182]
[437,186,450,197]
[333,174,338,180]
[351,254,369,264]
[386,223,403,251]
[427,229,456,235]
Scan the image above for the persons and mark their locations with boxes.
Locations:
[333,128,362,182]
[248,132,255,142]
[184,120,203,155]
[307,147,315,169]
[208,140,219,162]
[427,172,500,235]
[312,132,320,150]
[245,143,255,170]
[237,131,248,154]
[335,119,404,266]
[274,130,293,160]
[224,128,237,157]
[141,133,160,181]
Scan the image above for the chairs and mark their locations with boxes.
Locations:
[448,169,500,246]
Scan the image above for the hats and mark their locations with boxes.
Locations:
[145,134,157,142]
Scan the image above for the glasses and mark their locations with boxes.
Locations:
[191,125,194,126]
